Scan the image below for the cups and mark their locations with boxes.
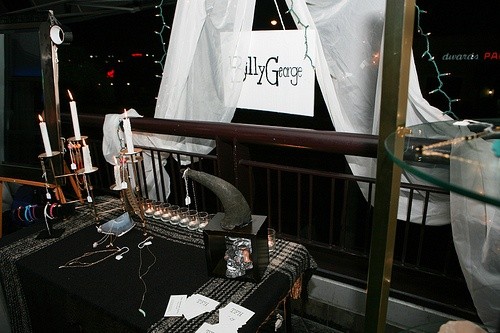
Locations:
[198,212,215,232]
[143,199,154,218]
[168,205,180,225]
[152,201,162,220]
[160,202,171,222]
[187,210,199,230]
[179,208,189,228]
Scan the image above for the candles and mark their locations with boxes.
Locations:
[39,114,53,157]
[82,138,93,171]
[67,88,81,141]
[112,155,122,189]
[122,108,136,153]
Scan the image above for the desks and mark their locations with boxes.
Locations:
[0,195,317,333]
[383,119,500,209]
